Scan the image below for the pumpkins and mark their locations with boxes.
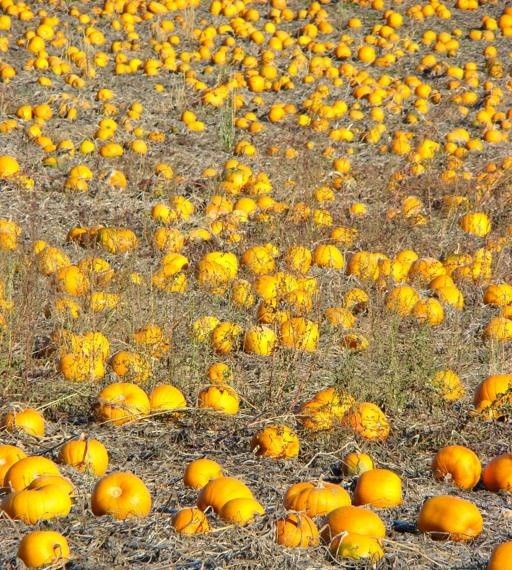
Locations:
[1,0,512,570]
